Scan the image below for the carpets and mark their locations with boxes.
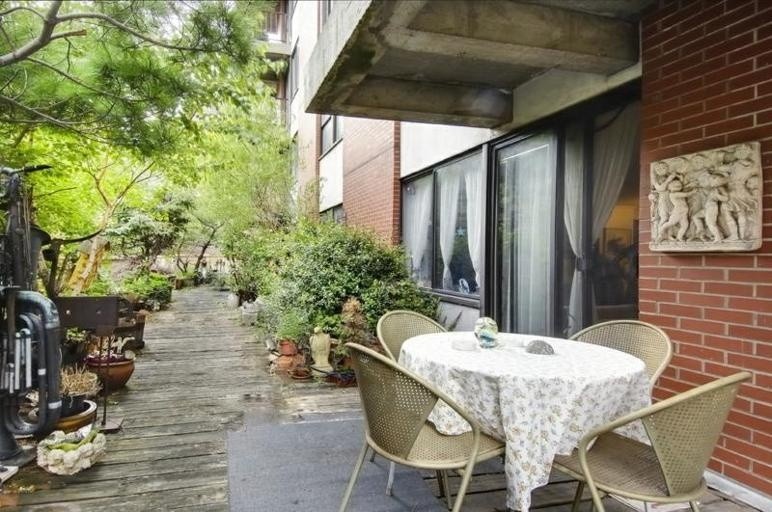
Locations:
[226,419,447,512]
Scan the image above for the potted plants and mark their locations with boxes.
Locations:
[28,367,102,429]
[84,354,135,392]
[277,318,302,356]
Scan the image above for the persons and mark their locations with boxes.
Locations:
[650,143,760,243]
